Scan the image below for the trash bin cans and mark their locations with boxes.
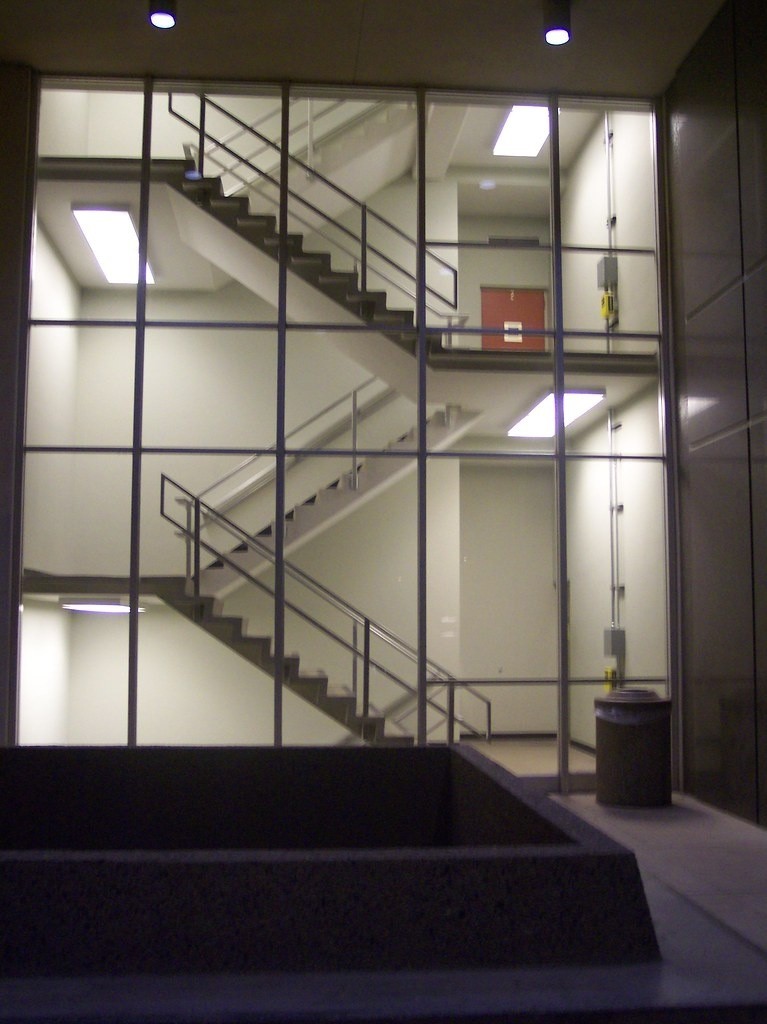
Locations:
[594,688,672,807]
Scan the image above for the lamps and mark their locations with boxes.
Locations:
[57,598,146,613]
[543,0,570,45]
[504,387,606,439]
[490,106,562,158]
[148,0,176,29]
[70,201,156,286]
